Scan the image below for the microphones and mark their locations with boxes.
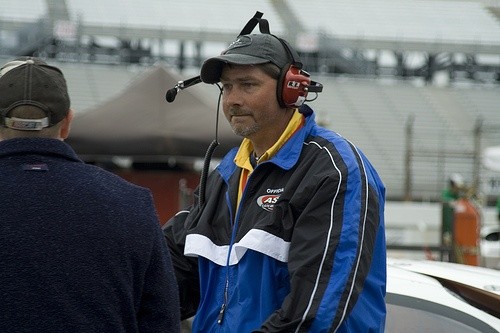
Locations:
[165,76,202,103]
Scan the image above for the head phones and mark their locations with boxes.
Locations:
[237,11,323,110]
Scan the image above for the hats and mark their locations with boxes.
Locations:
[0,57,70,131]
[200,33,303,84]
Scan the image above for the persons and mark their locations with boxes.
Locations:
[0,56,182,332]
[158,32,387,332]
[439,172,465,263]
